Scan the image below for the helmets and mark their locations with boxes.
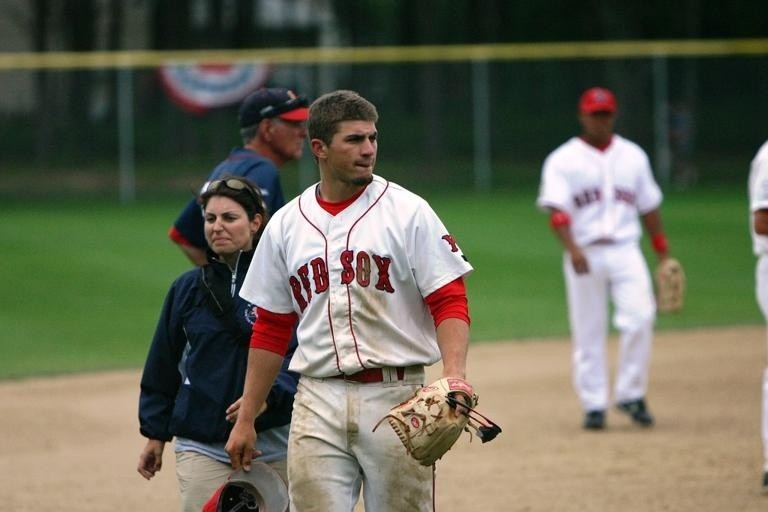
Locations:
[581,88,617,113]
[202,459,289,512]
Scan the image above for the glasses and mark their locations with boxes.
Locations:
[259,92,310,118]
[206,176,258,207]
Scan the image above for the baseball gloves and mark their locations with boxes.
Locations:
[655,258,687,314]
[375,374,501,465]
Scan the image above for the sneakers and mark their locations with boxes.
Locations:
[584,400,651,430]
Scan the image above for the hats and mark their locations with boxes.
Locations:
[238,88,310,129]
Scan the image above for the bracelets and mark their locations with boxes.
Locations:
[652,235,670,254]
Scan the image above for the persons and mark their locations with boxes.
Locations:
[137,173,300,512]
[223,88,475,512]
[167,87,309,267]
[746,141,768,488]
[535,86,668,431]
